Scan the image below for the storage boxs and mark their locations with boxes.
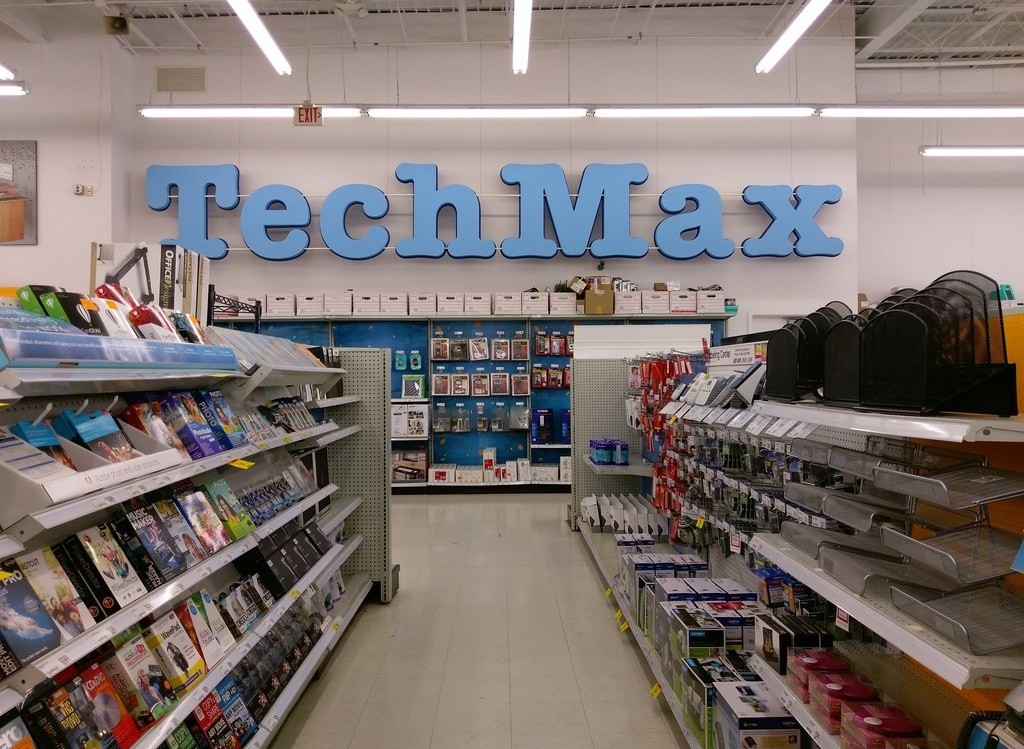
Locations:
[533,408,571,482]
[0,280,332,749]
[427,446,529,485]
[390,374,426,483]
[0,240,352,748]
[210,272,738,317]
[578,436,885,749]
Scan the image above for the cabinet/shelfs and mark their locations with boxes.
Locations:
[749,394,1024,749]
[531,444,572,485]
[390,397,428,488]
[0,367,372,749]
[576,454,657,547]
